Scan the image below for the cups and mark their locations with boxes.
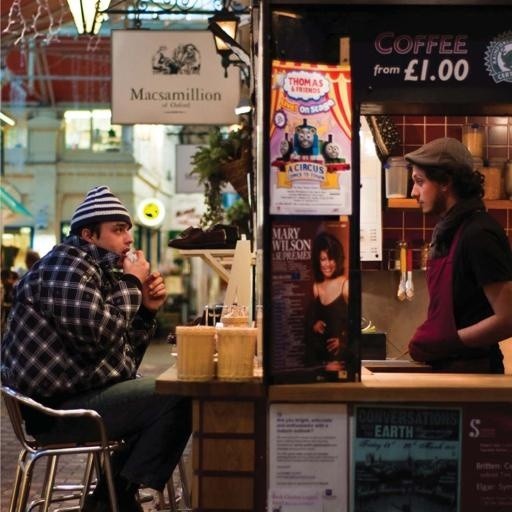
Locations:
[460,123,485,163]
[213,327,258,380]
[174,326,215,381]
[384,160,409,199]
[220,316,248,327]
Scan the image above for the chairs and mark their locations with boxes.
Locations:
[0,380,190,512]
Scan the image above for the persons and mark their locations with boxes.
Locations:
[6,272,19,305]
[24,251,41,269]
[404,139,512,372]
[1,185,192,511]
[305,230,348,363]
[2,271,14,307]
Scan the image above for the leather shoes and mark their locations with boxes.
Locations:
[169,226,228,247]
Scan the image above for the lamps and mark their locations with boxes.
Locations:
[208,8,251,81]
[66,0,251,35]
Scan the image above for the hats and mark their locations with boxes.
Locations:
[404,134,476,172]
[71,183,132,230]
